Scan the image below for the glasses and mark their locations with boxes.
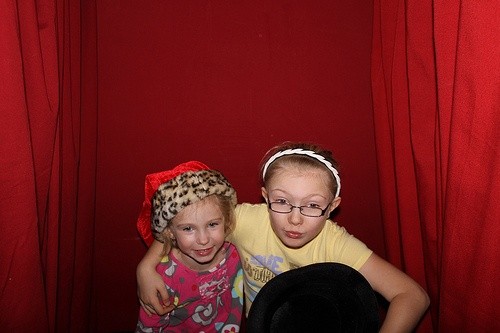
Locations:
[267,194,336,217]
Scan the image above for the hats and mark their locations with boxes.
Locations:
[137,160,237,249]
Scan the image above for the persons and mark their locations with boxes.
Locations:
[136,142,431,333]
[135,162,244,333]
